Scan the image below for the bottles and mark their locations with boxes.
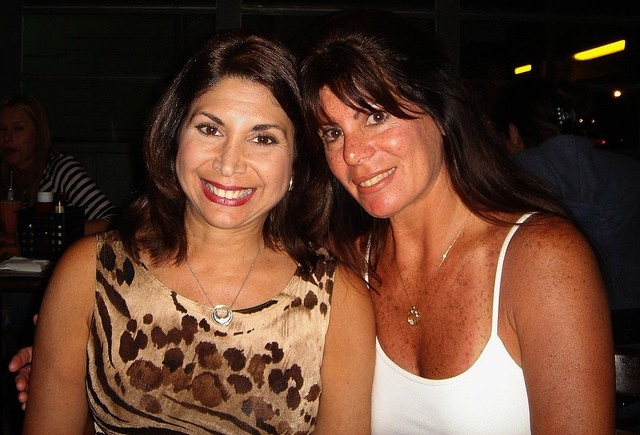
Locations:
[34,193,53,212]
[6,167,20,200]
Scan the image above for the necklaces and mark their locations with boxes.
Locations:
[393,212,470,326]
[184,240,265,325]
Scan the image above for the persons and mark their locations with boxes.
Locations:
[0,8,640,435]
